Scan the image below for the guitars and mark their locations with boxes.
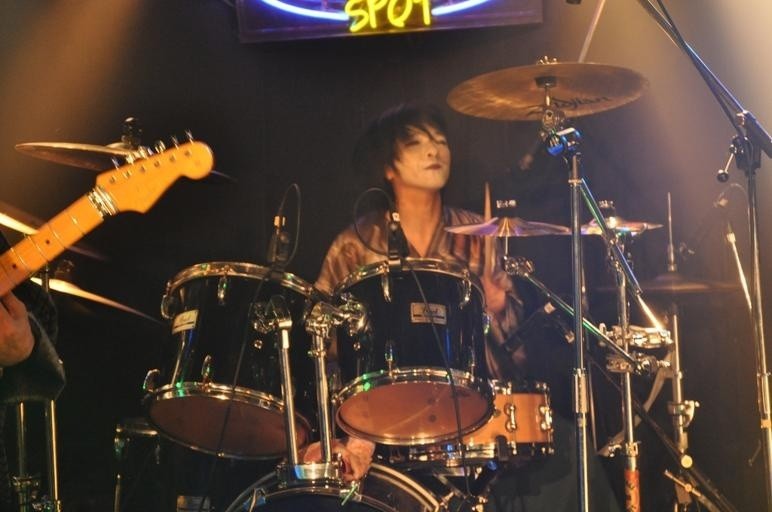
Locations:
[1,130,212,296]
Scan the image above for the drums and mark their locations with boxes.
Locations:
[393,380,557,472]
[225,458,479,512]
[144,262,328,461]
[334,257,496,446]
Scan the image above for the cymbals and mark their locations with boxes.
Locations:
[445,219,572,237]
[581,218,663,234]
[14,142,233,182]
[595,276,741,291]
[448,62,648,121]
[0,203,103,262]
[31,276,157,323]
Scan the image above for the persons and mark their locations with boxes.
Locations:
[0,230,66,404]
[313,102,528,378]
